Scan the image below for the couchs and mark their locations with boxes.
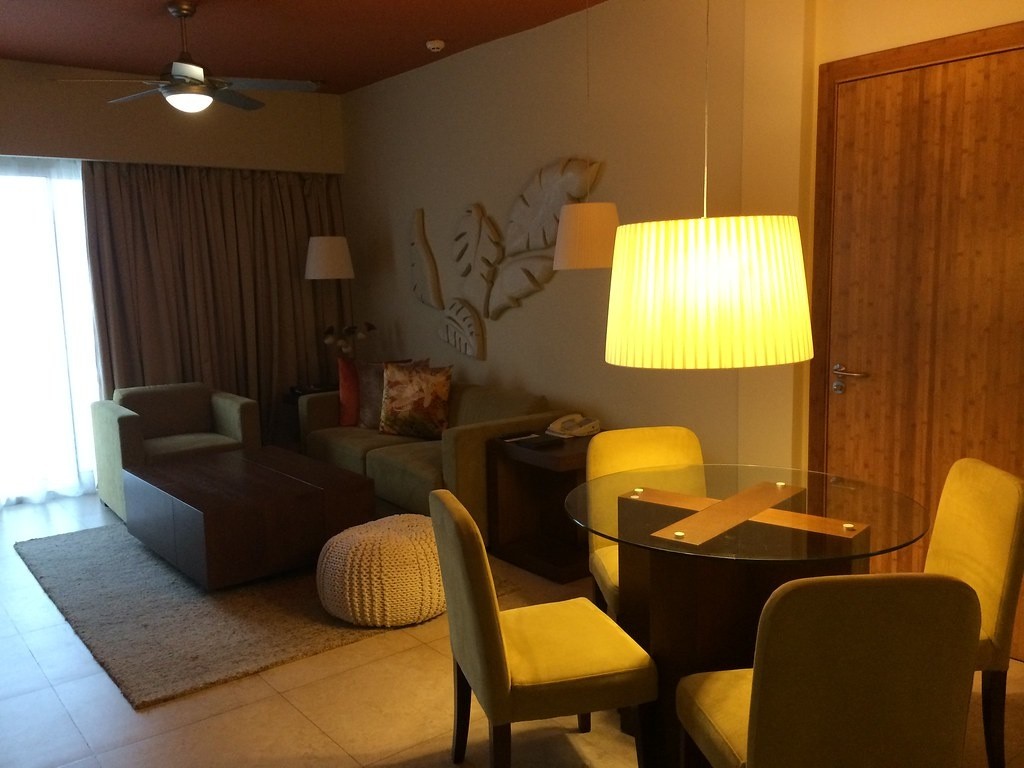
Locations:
[92,381,259,525]
[298,384,583,548]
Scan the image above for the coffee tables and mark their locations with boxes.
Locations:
[565,465,930,737]
[122,444,375,590]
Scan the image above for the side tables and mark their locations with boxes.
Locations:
[484,428,606,585]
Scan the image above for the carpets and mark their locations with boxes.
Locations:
[9,524,519,712]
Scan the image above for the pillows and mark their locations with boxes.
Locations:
[378,361,455,440]
[354,357,430,428]
[337,356,413,427]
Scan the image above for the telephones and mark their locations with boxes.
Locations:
[549,413,600,436]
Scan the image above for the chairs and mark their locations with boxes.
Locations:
[427,489,657,768]
[585,425,707,621]
[677,573,980,768]
[923,458,1024,768]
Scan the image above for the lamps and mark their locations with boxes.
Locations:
[608,2,812,370]
[304,235,356,356]
[48,1,328,114]
[551,1,620,272]
